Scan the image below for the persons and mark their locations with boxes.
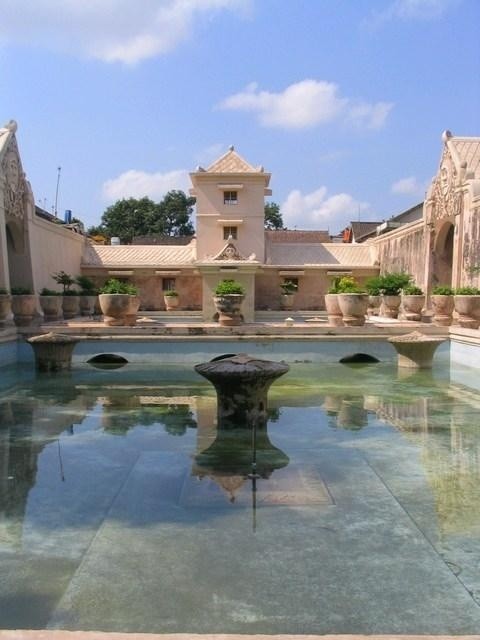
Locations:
[438,158,455,198]
[214,243,246,262]
[2,152,20,194]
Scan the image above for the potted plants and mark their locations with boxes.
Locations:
[324,271,480,329]
[163,290,181,310]
[0,269,141,327]
[279,279,298,310]
[212,279,246,326]
